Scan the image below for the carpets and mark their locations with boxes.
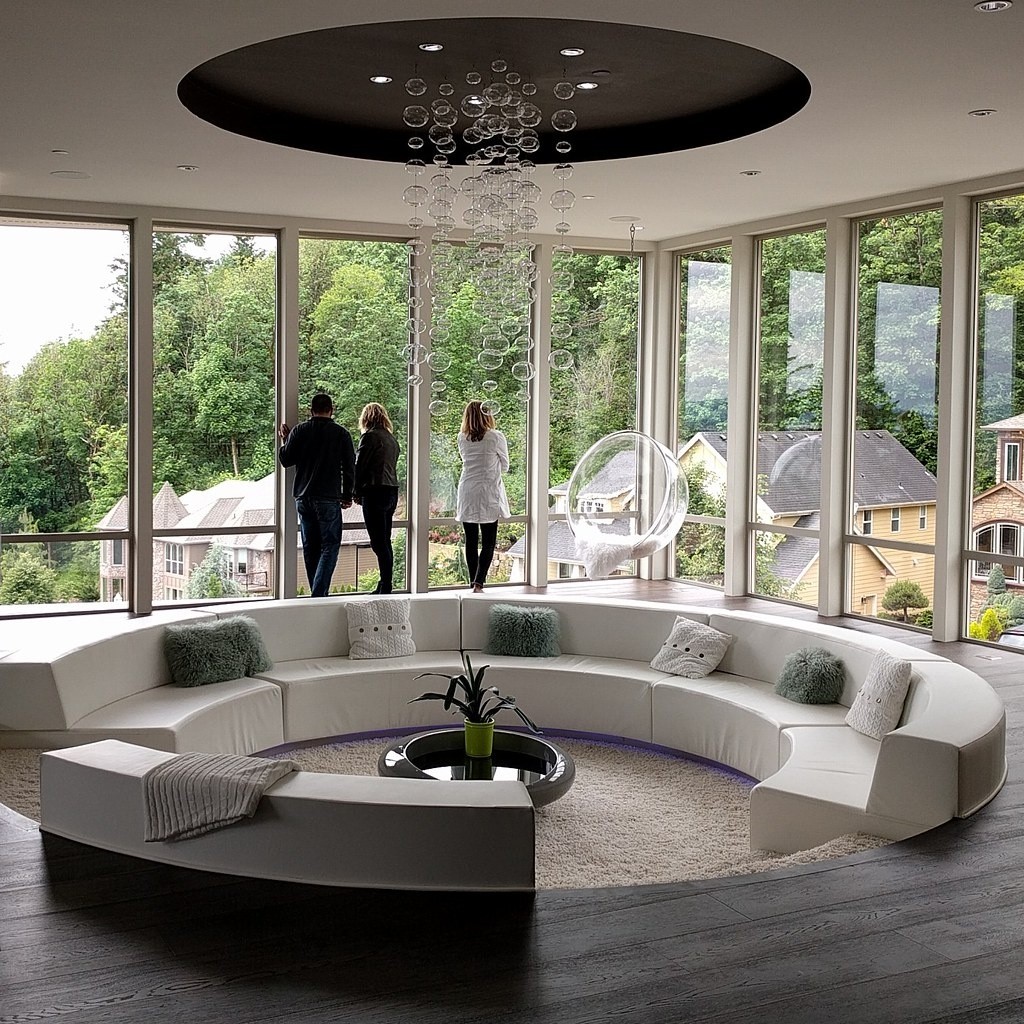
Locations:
[0,735,897,889]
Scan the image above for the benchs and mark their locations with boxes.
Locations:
[0,592,1008,888]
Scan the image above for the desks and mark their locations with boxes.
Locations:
[378,728,576,808]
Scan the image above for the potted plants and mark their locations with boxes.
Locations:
[406,654,544,756]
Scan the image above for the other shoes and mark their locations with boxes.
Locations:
[371,581,392,595]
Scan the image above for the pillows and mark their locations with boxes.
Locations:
[650,614,732,679]
[845,648,912,741]
[160,613,274,688]
[344,597,416,660]
[773,647,848,705]
[480,602,561,658]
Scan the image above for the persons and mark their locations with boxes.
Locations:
[454,401,512,593]
[278,394,356,598]
[353,402,401,594]
